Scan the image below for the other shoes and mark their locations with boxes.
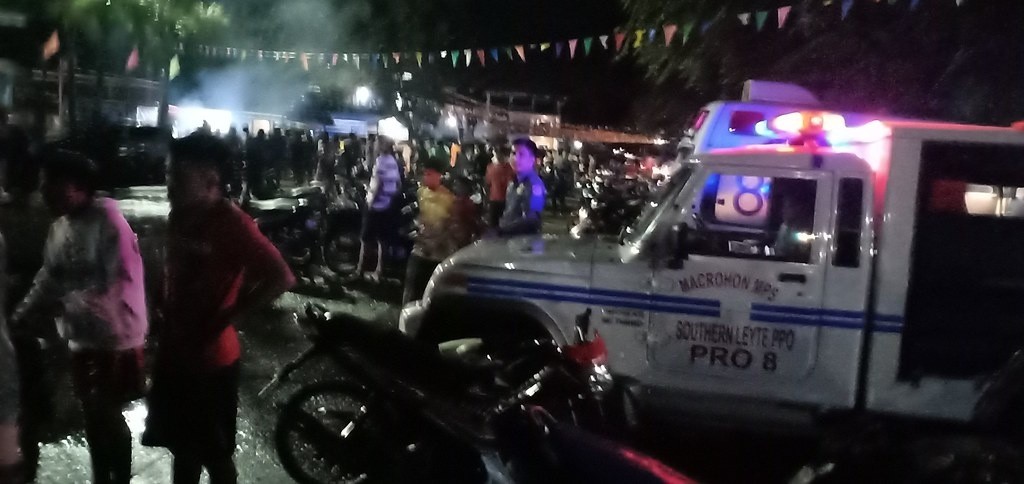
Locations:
[364,273,381,285]
[346,271,364,282]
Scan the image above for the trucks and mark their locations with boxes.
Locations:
[400,80,1024,447]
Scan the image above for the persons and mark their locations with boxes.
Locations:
[9,151,150,484]
[766,185,815,261]
[139,120,652,326]
[146,132,294,484]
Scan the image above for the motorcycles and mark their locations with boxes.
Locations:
[254,301,637,484]
[562,146,652,239]
[229,178,356,275]
